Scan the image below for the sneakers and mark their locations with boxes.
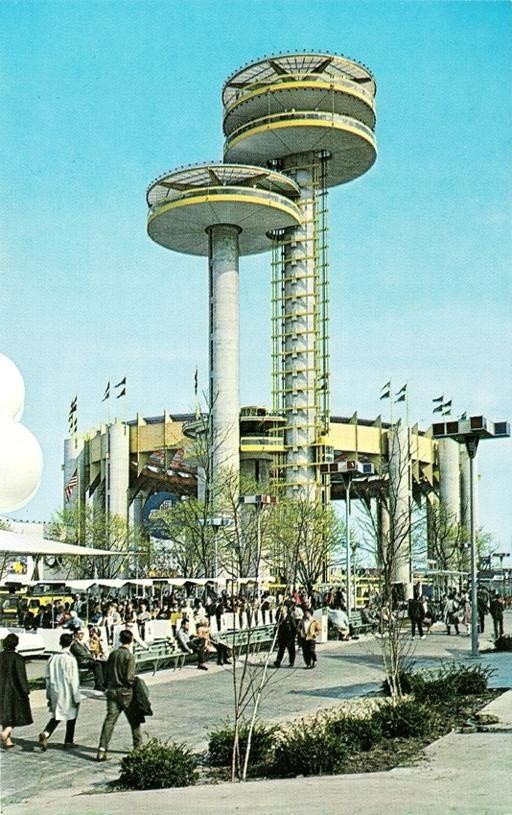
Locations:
[218,661,232,665]
[39,733,47,749]
[307,661,317,668]
[63,743,79,749]
[97,752,111,760]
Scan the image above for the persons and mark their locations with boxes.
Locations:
[0,633,36,752]
[400,585,512,640]
[1,584,400,692]
[39,633,83,753]
[95,630,145,762]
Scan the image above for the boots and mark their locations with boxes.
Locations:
[448,626,459,634]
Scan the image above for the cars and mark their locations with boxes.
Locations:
[393,601,410,620]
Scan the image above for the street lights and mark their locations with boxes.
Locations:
[426,542,511,610]
[196,517,236,580]
[337,459,377,616]
[347,539,362,613]
[238,493,282,626]
[429,412,512,657]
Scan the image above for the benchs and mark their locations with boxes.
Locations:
[327,609,399,640]
[79,610,305,676]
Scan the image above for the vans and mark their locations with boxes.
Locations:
[1,555,78,629]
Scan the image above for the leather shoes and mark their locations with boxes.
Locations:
[274,662,280,668]
[0,734,15,748]
[198,665,208,670]
[352,637,359,639]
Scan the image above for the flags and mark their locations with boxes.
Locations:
[62,466,79,505]
[379,381,467,422]
[68,378,125,437]
